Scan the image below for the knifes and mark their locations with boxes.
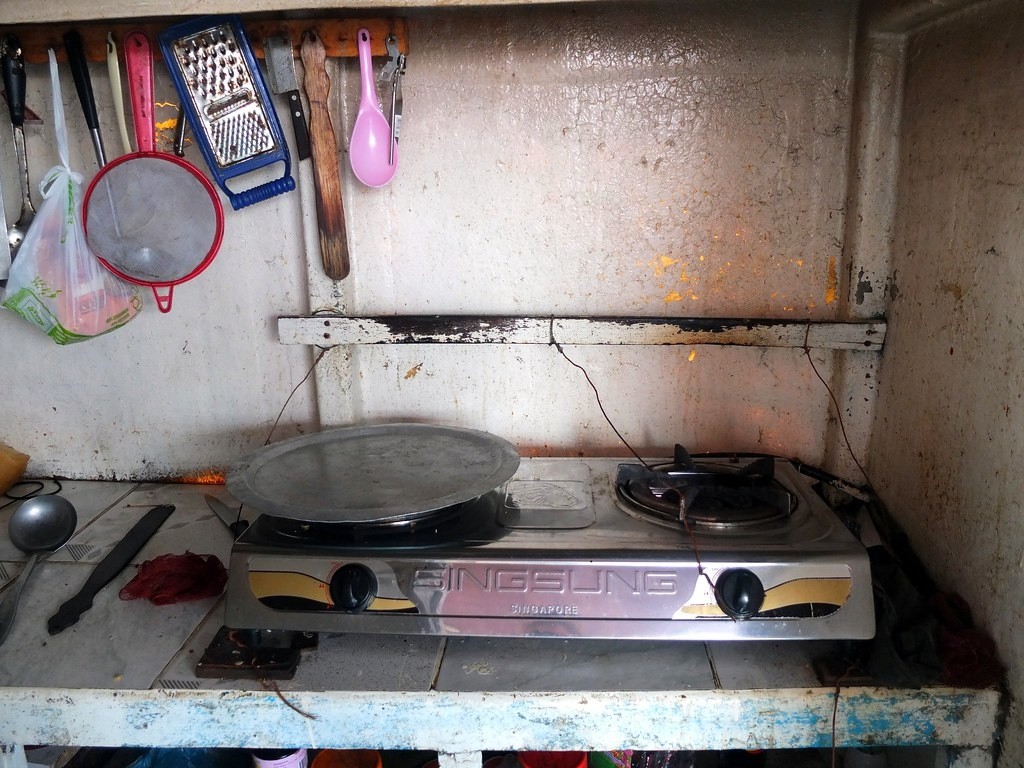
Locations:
[205,491,249,540]
[262,32,311,161]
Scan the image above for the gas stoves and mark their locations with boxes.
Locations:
[222,443,876,641]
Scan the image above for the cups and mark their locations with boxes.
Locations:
[311,750,382,768]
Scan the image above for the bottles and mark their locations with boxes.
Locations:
[250,748,308,768]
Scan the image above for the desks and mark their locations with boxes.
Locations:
[0,477,1005,768]
